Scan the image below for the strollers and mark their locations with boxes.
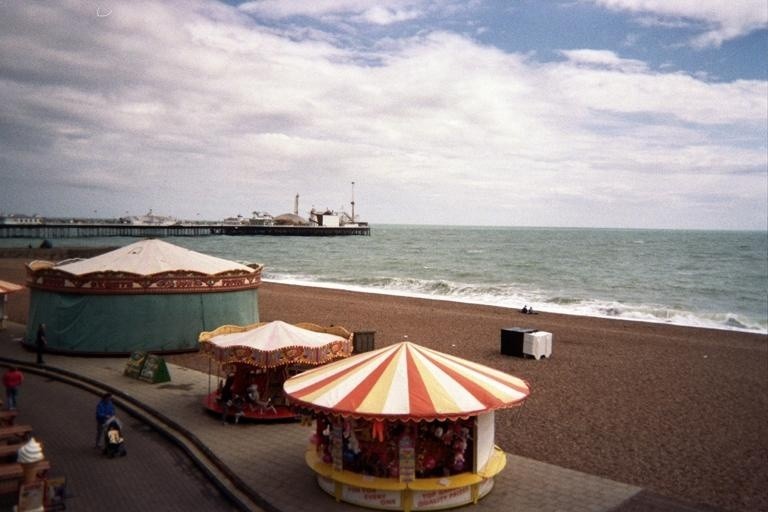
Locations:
[100,415,128,457]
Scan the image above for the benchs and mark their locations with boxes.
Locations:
[0,398,50,512]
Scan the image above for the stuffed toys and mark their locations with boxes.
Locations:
[301,409,474,480]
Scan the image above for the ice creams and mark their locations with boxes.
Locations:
[17,438,45,482]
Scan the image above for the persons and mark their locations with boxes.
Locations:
[1,364,25,411]
[107,426,122,444]
[94,392,117,454]
[521,305,528,314]
[529,306,534,314]
[34,321,48,364]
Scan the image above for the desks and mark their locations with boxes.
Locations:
[522,330,552,360]
[501,326,538,358]
